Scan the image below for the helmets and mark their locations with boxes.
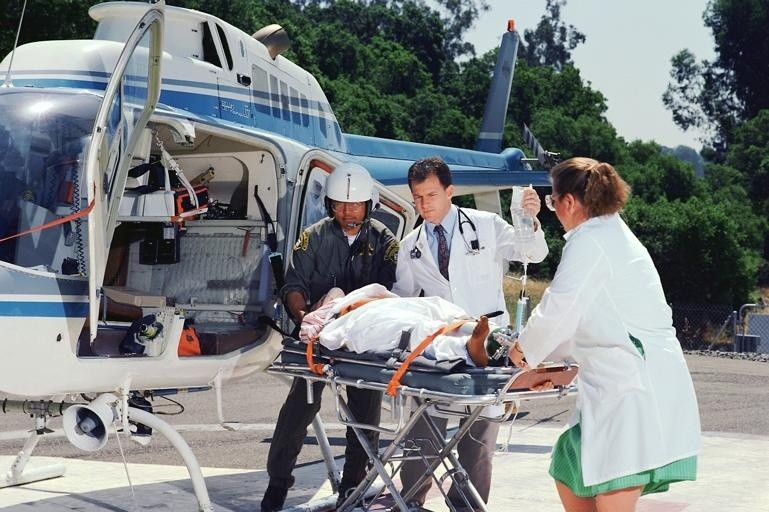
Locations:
[324,163,380,219]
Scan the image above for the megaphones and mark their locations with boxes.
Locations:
[61,393,121,452]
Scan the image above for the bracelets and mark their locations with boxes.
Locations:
[515,343,523,354]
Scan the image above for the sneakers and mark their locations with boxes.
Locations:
[337,471,367,509]
[261,475,294,512]
[391,500,422,511]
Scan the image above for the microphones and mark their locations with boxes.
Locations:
[346,204,380,228]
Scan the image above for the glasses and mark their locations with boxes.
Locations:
[544,190,568,212]
[330,201,367,213]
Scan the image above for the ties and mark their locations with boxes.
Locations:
[435,225,450,281]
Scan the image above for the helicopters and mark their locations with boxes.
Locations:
[0,0,563,512]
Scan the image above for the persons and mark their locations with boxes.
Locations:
[507,156,702,512]
[259,162,400,510]
[296,282,526,368]
[387,155,550,511]
